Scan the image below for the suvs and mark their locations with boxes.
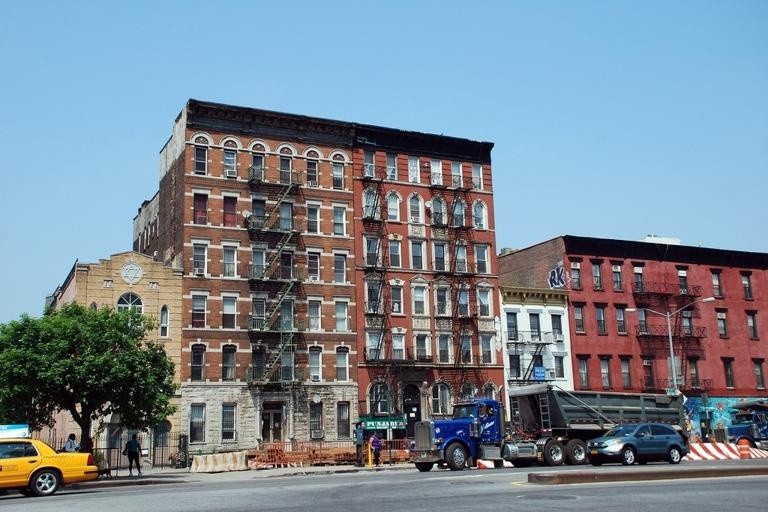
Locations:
[585,422,687,466]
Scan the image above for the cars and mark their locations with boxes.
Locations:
[0,424,100,496]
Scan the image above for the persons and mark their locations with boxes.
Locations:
[369,431,381,468]
[64,433,79,452]
[123,435,144,477]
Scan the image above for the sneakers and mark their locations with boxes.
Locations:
[129,474,133,476]
[138,472,143,477]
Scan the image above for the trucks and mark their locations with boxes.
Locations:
[705,399,768,451]
[408,382,691,472]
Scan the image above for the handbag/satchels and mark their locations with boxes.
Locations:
[122,448,130,455]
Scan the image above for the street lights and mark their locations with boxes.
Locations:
[625,297,714,444]
